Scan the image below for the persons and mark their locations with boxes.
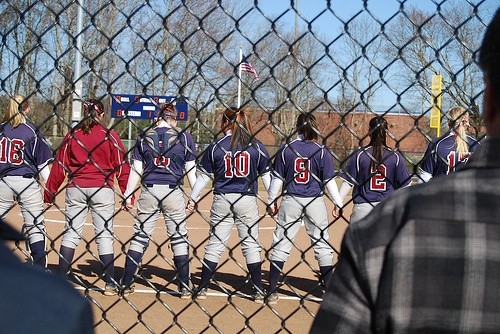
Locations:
[265,112,344,305]
[185,107,277,303]
[43,99,134,295]
[117,104,201,299]
[309,5,500,334]
[333,115,413,221]
[418,107,482,184]
[0,218,95,334]
[0,94,56,271]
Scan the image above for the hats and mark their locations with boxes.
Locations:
[477,7,500,71]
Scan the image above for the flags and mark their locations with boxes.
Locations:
[241,53,259,82]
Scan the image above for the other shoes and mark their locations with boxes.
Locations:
[255,292,264,303]
[104,285,119,295]
[307,290,325,301]
[119,287,135,299]
[180,290,192,299]
[197,288,208,300]
[268,292,279,305]
